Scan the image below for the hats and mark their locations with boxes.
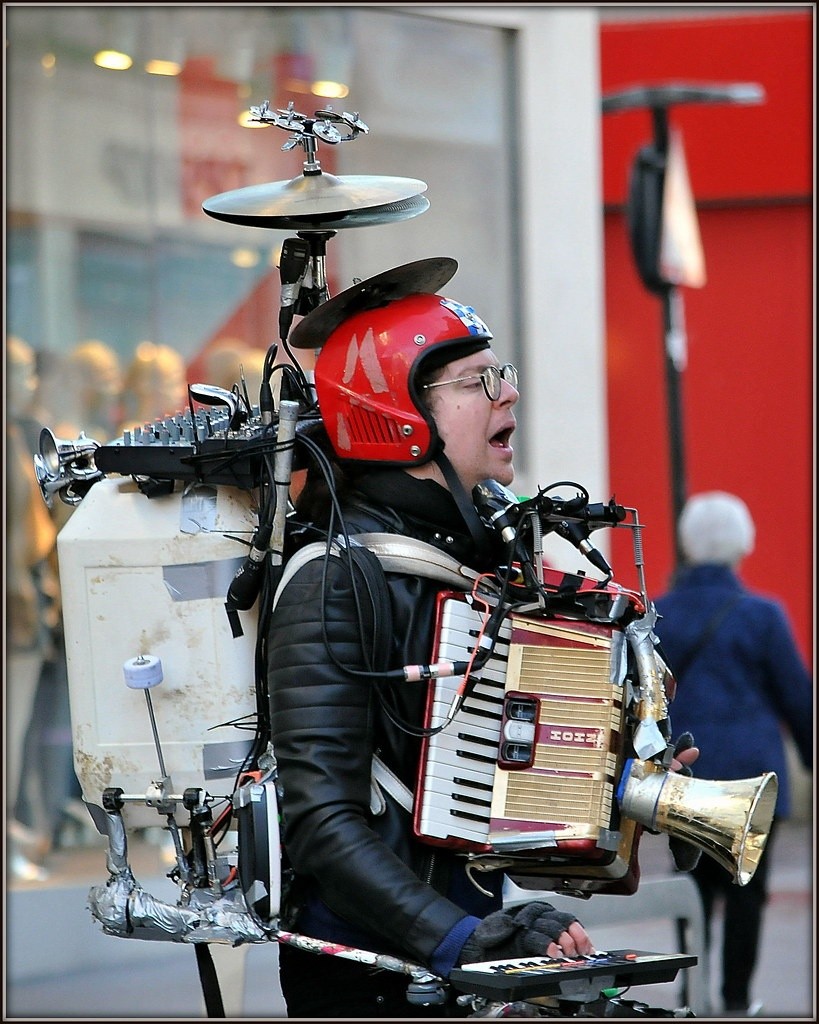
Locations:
[679,489,753,563]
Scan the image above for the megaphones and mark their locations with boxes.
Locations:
[616,757,778,886]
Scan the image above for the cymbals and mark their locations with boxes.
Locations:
[196,167,435,232]
[286,254,460,349]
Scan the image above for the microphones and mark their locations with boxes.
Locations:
[552,521,613,573]
[226,522,274,611]
[276,239,311,339]
[471,480,531,572]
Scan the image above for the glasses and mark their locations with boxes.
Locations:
[422,363,519,401]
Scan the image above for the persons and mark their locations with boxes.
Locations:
[268,292,701,1023]
[14,549,107,858]
[647,489,811,1009]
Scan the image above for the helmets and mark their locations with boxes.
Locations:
[315,292,494,466]
